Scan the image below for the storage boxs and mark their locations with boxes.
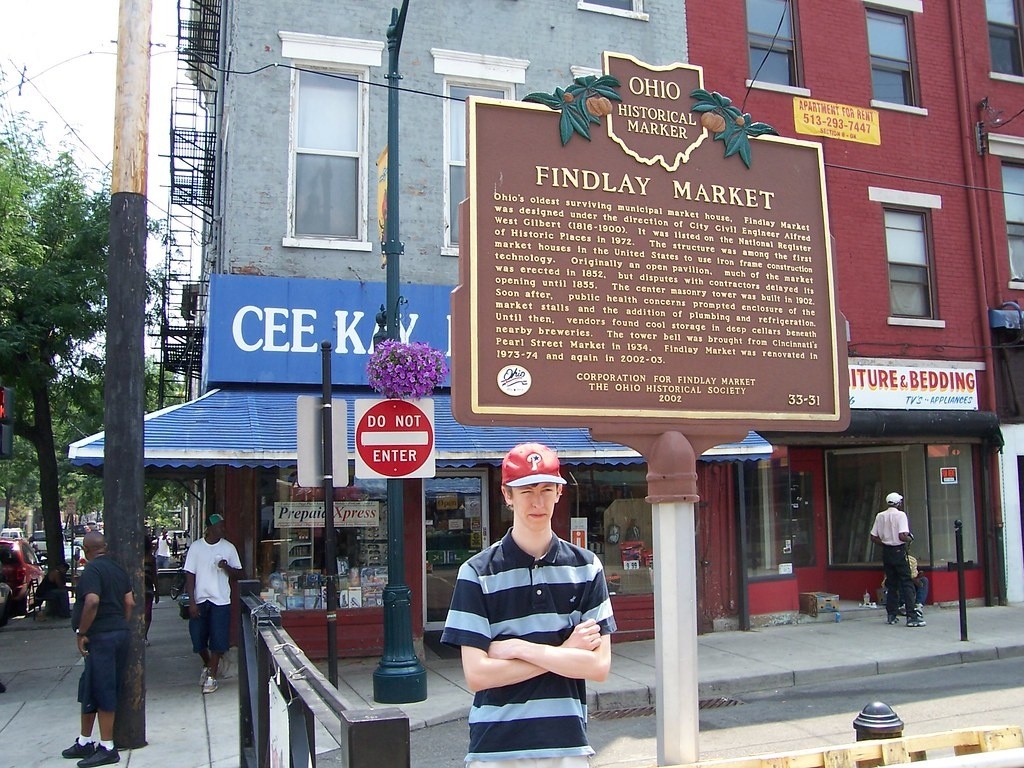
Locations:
[800,592,839,613]
[287,596,304,610]
[305,596,321,609]
[349,587,362,607]
[337,557,350,576]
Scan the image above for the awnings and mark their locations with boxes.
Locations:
[67,385,775,481]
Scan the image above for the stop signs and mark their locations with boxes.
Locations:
[355,399,435,478]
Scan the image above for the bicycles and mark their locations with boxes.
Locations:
[167,552,187,600]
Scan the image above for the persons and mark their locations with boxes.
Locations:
[36,563,72,619]
[184,513,242,693]
[145,536,159,646]
[155,524,192,569]
[881,554,928,618]
[871,492,927,626]
[439,442,617,768]
[62,531,135,768]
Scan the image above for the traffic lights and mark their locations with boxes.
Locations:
[0,386,12,459]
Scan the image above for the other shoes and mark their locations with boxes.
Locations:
[914,603,924,617]
[197,666,211,685]
[886,615,900,624]
[0,682,6,693]
[906,616,927,627]
[144,639,151,647]
[203,676,219,694]
[898,605,906,616]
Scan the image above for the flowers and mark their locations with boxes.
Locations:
[367,338,450,402]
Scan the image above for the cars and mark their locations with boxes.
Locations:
[0,561,13,625]
[0,538,47,615]
[1,520,187,561]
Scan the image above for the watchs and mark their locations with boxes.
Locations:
[76,628,86,636]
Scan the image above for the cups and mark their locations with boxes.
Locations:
[863,595,870,605]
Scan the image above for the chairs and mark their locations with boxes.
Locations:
[31,577,56,621]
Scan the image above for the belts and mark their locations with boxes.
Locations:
[884,543,906,548]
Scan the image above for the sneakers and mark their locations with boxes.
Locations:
[62,737,97,759]
[77,743,121,768]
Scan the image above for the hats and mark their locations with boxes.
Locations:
[886,493,903,506]
[205,513,224,527]
[501,442,569,488]
